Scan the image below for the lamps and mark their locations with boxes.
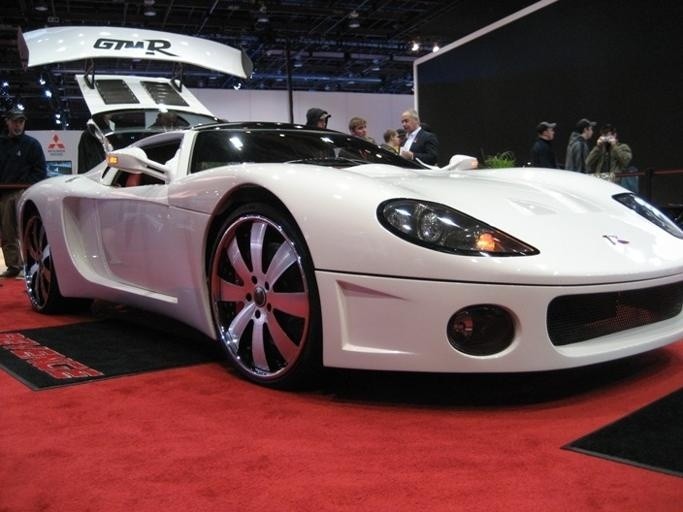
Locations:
[142,0,158,18]
[257,7,269,24]
[346,9,360,29]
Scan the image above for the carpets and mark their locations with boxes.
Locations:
[556,383,683,484]
[0,307,233,392]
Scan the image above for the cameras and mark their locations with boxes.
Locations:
[600,136,610,143]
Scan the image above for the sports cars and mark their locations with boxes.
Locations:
[13,26,682,386]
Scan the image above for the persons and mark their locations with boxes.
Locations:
[0,100,48,278]
[563,118,598,173]
[348,117,378,146]
[396,129,406,144]
[397,109,440,168]
[307,108,330,129]
[379,128,400,156]
[523,121,557,168]
[585,124,633,184]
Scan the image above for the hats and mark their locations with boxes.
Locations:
[6,108,27,122]
[320,112,331,120]
[576,119,598,132]
[536,122,557,132]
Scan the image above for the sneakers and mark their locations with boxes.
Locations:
[1,267,20,278]
